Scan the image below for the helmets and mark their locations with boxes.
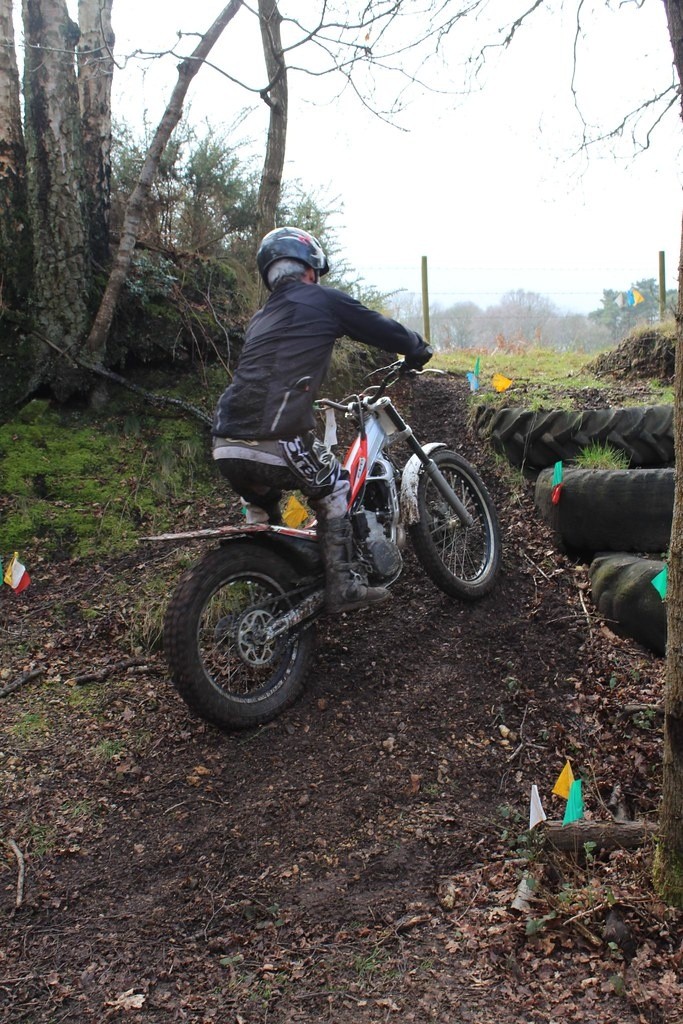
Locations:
[257,226,330,292]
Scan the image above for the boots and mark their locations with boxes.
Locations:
[317,519,392,615]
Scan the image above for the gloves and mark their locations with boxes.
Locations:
[409,341,432,372]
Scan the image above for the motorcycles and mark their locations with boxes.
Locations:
[130,342,503,731]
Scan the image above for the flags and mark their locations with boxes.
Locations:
[651,564,666,600]
[281,496,309,529]
[615,288,644,308]
[4,552,31,594]
[563,780,583,828]
[550,461,563,504]
[466,356,480,391]
[552,761,576,800]
[492,372,512,391]
[529,785,547,830]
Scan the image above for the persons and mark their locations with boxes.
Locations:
[212,227,433,612]
